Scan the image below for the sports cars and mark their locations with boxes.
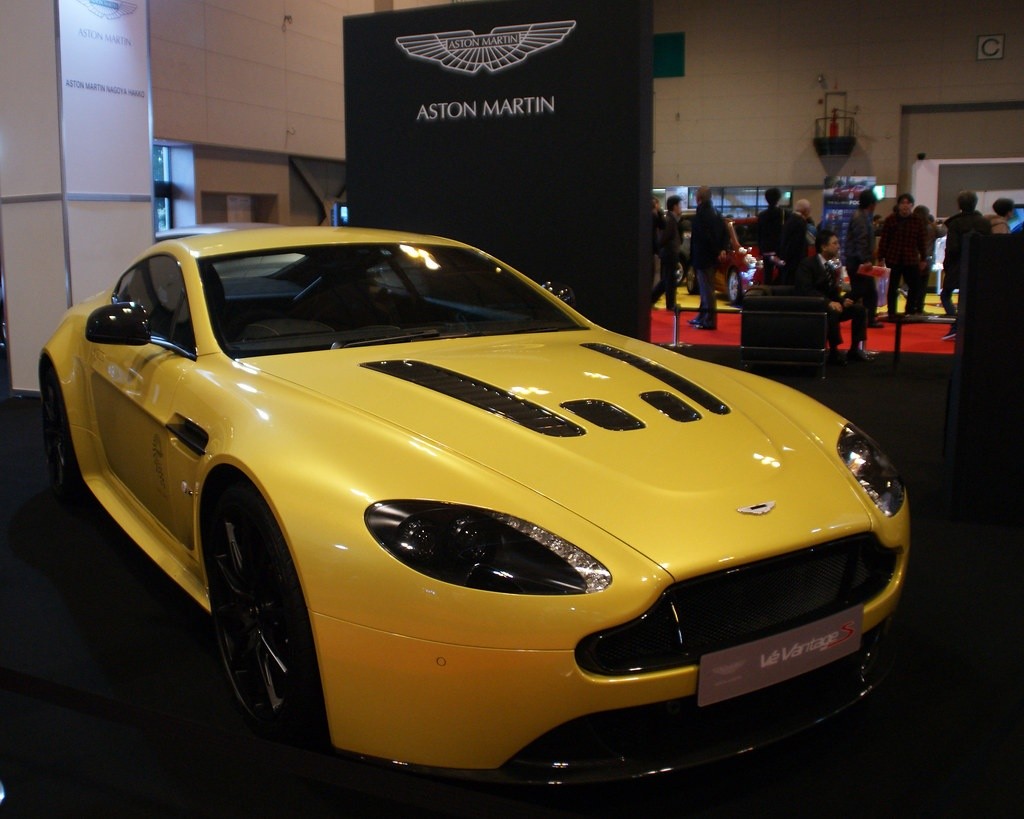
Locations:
[37,221,908,790]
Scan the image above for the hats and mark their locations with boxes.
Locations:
[860,191,876,202]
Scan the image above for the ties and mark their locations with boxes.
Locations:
[825,263,833,288]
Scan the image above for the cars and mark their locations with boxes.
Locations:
[664,207,843,305]
[833,183,868,200]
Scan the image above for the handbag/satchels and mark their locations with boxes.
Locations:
[877,264,889,306]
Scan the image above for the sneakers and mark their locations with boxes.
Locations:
[942,325,957,339]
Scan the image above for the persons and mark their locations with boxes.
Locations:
[756,184,1024,365]
[689,185,730,330]
[650,194,685,312]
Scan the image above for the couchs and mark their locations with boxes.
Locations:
[742,284,831,380]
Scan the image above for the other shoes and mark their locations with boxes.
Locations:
[696,323,717,328]
[847,349,874,362]
[831,352,844,361]
[687,319,699,325]
[868,319,882,327]
